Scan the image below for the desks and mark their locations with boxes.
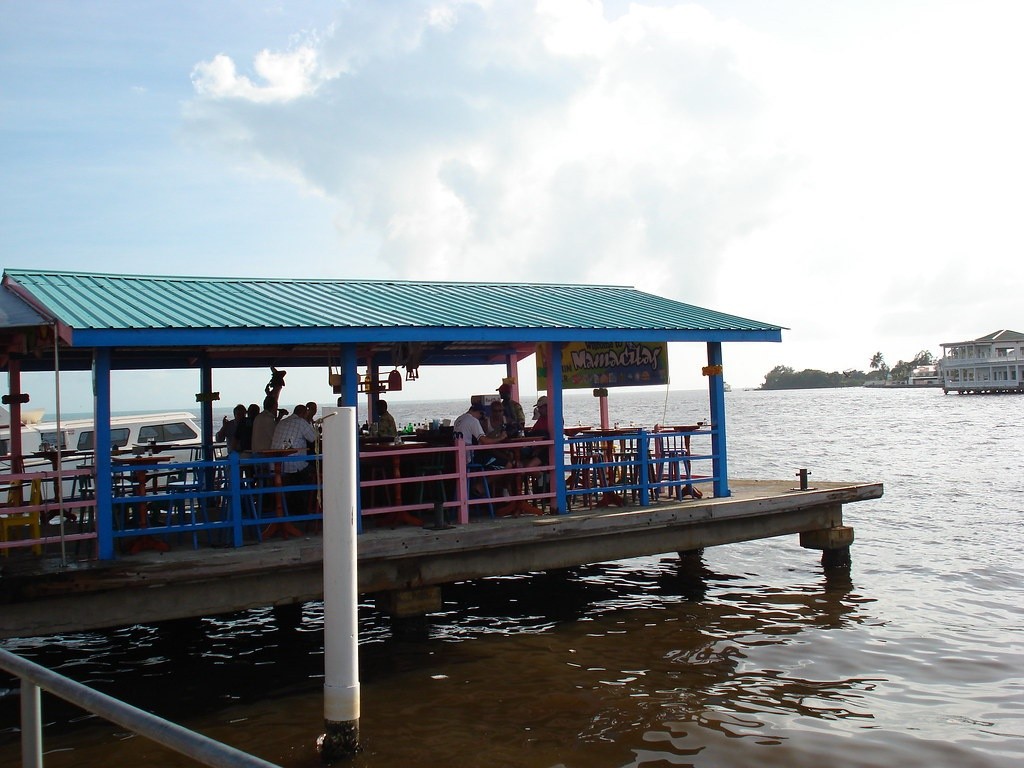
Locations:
[113,455,174,556]
[583,427,637,507]
[370,440,429,530]
[563,425,593,486]
[496,436,546,517]
[252,445,309,540]
[661,424,703,499]
[32,448,77,523]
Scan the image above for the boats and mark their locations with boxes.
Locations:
[0,405,215,517]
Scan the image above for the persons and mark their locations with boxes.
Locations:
[453,384,548,498]
[215,396,322,486]
[375,400,397,433]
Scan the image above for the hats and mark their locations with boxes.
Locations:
[532,407,540,420]
[533,396,547,407]
[496,384,511,393]
[270,365,286,386]
[472,403,486,415]
[491,401,505,410]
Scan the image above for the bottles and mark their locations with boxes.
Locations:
[630,421,635,428]
[614,422,620,430]
[579,421,581,427]
[147,437,154,446]
[704,418,707,425]
[394,435,402,445]
[398,419,428,433]
[39,442,55,452]
[145,449,152,458]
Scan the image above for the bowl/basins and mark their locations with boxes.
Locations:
[697,422,703,425]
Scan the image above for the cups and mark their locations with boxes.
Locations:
[433,420,440,431]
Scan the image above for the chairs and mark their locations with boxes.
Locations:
[0,422,703,560]
